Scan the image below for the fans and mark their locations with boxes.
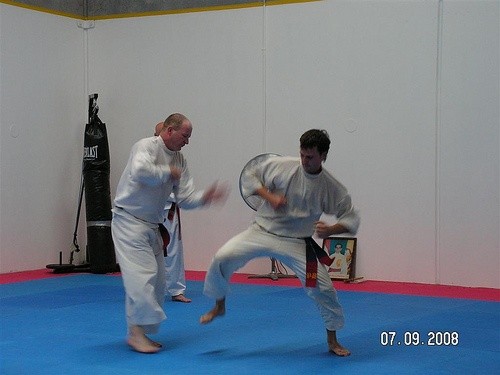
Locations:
[237,153,293,282]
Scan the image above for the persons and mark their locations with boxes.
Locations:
[151,121,192,304]
[197,129,361,358]
[110,114,229,353]
[325,243,348,276]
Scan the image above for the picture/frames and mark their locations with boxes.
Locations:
[321,236,357,282]
